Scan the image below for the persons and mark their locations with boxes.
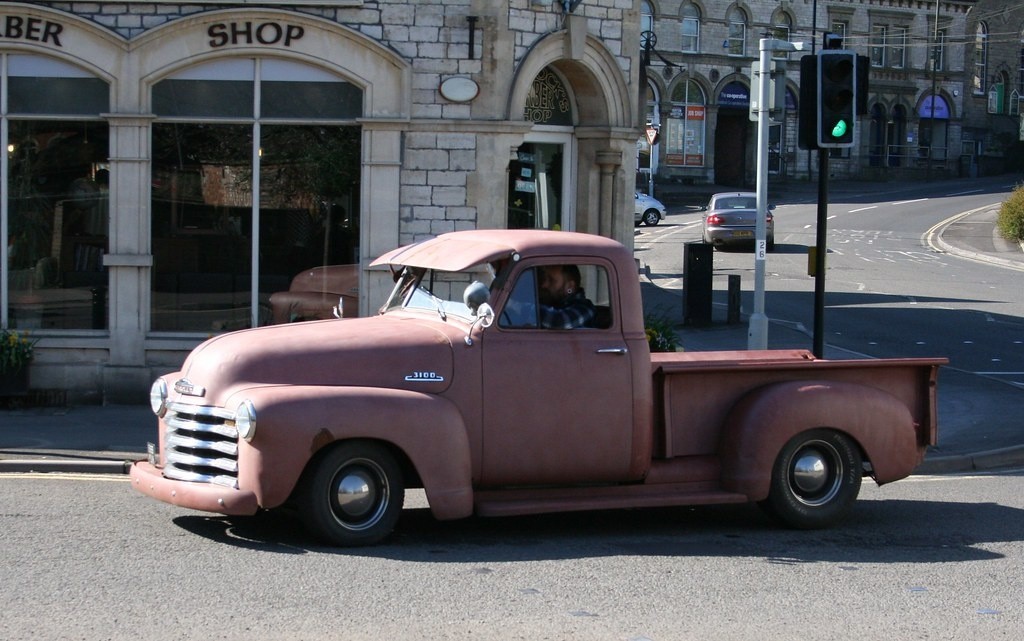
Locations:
[66,169,108,237]
[331,204,345,235]
[508,265,595,330]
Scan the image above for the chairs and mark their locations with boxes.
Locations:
[591,305,613,328]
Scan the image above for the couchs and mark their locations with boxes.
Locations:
[268,263,360,325]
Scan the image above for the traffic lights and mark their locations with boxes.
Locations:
[817,49,856,148]
[820,31,845,51]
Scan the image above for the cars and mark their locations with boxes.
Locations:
[635,190,667,226]
[698,191,776,251]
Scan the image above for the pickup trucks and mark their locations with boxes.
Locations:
[265,260,358,324]
[129,228,950,549]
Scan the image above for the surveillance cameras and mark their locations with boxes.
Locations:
[826,34,842,49]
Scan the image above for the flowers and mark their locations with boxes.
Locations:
[0,321,43,375]
